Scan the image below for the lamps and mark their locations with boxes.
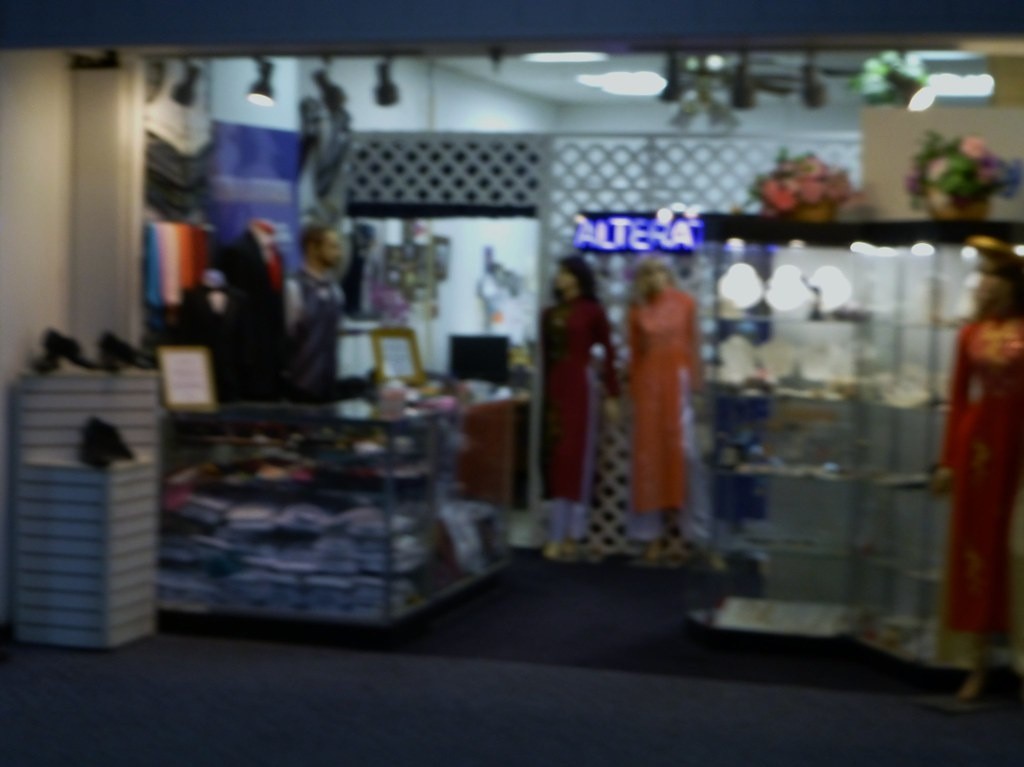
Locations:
[310,57,347,110]
[799,53,827,108]
[247,56,275,107]
[887,70,937,112]
[170,57,199,106]
[374,56,401,106]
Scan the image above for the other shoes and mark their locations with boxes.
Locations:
[77,416,131,470]
[97,330,158,370]
[45,328,121,374]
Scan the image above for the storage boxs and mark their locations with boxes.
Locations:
[5,369,158,647]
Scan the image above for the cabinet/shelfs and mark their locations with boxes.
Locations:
[701,305,881,669]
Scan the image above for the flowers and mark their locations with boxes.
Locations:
[904,130,1024,211]
[731,147,864,217]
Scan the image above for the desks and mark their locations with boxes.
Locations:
[157,384,528,647]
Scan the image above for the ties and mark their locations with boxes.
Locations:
[266,242,282,289]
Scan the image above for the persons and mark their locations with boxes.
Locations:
[205,218,287,405]
[625,250,714,569]
[284,224,346,404]
[540,256,624,563]
[928,256,1023,712]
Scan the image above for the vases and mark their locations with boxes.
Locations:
[784,204,839,222]
[924,186,992,219]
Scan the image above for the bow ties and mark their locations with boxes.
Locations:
[317,278,332,291]
